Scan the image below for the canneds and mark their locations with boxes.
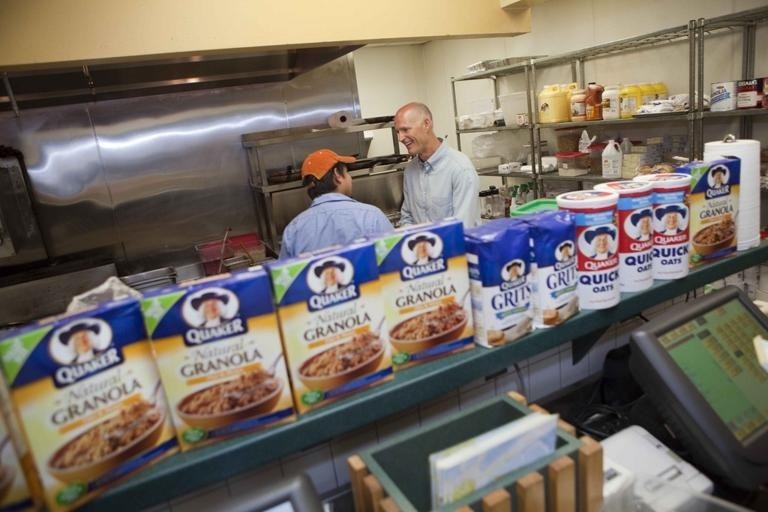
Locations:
[737,80,757,110]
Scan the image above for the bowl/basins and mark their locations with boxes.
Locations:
[391,311,466,356]
[174,377,285,432]
[692,221,735,255]
[298,339,386,394]
[46,406,165,484]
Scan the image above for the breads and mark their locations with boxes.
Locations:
[542,309,560,325]
[488,329,504,347]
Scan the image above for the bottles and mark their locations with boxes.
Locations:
[569,81,668,122]
[479,185,511,219]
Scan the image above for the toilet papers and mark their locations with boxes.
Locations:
[702,138,762,252]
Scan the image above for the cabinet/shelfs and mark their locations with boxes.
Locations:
[698,5,766,168]
[450,51,577,220]
[531,10,749,202]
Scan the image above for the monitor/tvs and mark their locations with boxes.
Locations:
[627,284,768,492]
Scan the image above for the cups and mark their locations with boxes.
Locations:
[498,162,521,174]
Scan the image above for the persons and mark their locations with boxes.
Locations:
[655,205,687,235]
[278,148,395,259]
[59,323,102,364]
[507,263,522,281]
[192,294,231,327]
[559,244,572,261]
[394,102,482,230]
[585,226,616,259]
[408,236,436,265]
[631,210,653,241]
[315,261,347,293]
[712,167,726,188]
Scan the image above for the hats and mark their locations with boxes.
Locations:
[301,149,356,191]
[584,226,616,243]
[711,167,725,176]
[654,205,686,220]
[506,263,520,271]
[191,292,228,310]
[407,235,434,251]
[59,322,99,345]
[630,209,652,226]
[314,260,344,278]
[559,243,570,250]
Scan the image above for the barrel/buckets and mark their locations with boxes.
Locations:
[617,137,635,154]
[537,82,669,120]
[601,139,624,179]
